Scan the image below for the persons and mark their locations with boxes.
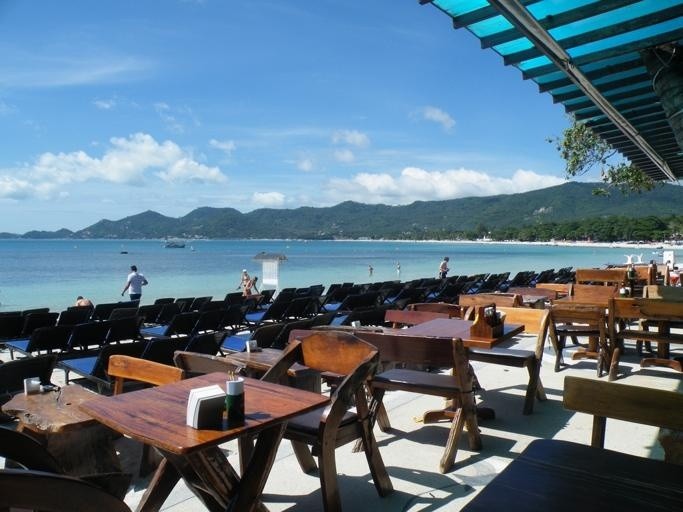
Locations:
[437,255,450,279]
[244,277,260,300]
[120,265,149,304]
[235,268,250,291]
[73,295,97,309]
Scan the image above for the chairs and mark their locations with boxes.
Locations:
[310,284,322,288]
[480,292,523,306]
[22,308,49,326]
[384,288,416,305]
[190,296,213,312]
[412,288,428,302]
[220,323,285,352]
[353,330,483,476]
[348,287,361,295]
[272,320,313,348]
[0,316,23,342]
[21,312,60,339]
[225,292,244,301]
[407,304,464,320]
[567,283,619,297]
[507,287,558,300]
[219,305,242,329]
[66,320,113,351]
[328,287,347,303]
[636,285,683,355]
[459,275,467,283]
[156,302,180,325]
[604,266,652,286]
[287,329,347,397]
[154,298,175,305]
[245,301,291,325]
[544,300,612,377]
[373,303,397,324]
[360,291,382,307]
[296,292,310,298]
[468,266,575,294]
[192,308,226,336]
[282,297,312,320]
[140,311,201,337]
[0,354,57,394]
[454,283,465,295]
[310,287,325,299]
[327,283,342,297]
[283,287,297,293]
[108,354,184,396]
[67,304,93,322]
[576,268,627,285]
[142,335,192,358]
[0,469,133,512]
[535,282,569,296]
[446,306,550,416]
[58,309,90,326]
[563,375,682,461]
[201,300,227,312]
[175,297,195,311]
[298,287,309,292]
[442,276,459,291]
[5,325,65,360]
[185,333,229,356]
[111,315,146,344]
[329,310,374,325]
[228,296,247,309]
[312,324,383,334]
[58,339,149,394]
[0,310,22,316]
[321,294,361,313]
[254,331,393,512]
[430,285,452,302]
[118,299,140,309]
[109,308,137,321]
[173,350,249,378]
[607,298,683,379]
[355,276,441,289]
[384,310,450,329]
[342,282,354,288]
[259,289,276,305]
[459,295,517,307]
[139,304,164,324]
[258,291,294,310]
[91,303,121,321]
